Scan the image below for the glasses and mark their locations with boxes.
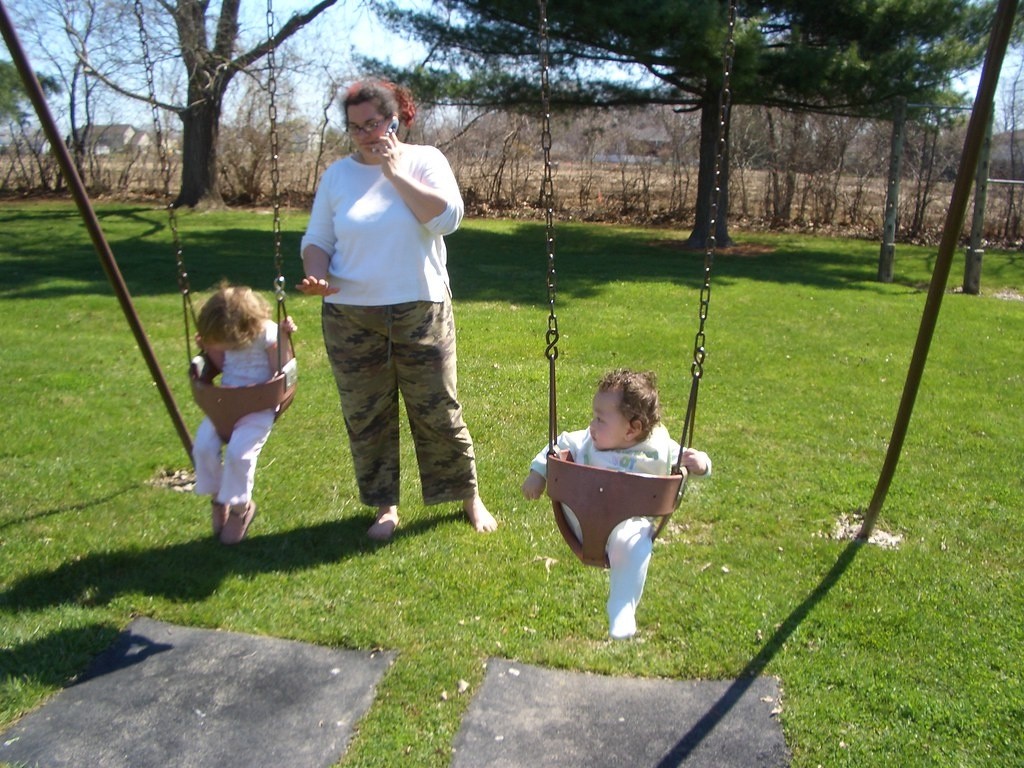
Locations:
[346,118,385,134]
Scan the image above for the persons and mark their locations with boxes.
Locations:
[523,370,712,640]
[192,286,299,545]
[297,82,498,540]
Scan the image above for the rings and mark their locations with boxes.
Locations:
[384,146,388,151]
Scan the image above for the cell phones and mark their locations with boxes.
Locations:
[373,118,399,158]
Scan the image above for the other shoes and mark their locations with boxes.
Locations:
[212,499,231,533]
[219,499,257,545]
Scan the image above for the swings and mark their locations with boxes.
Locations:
[129,1,301,449]
[536,2,739,570]
